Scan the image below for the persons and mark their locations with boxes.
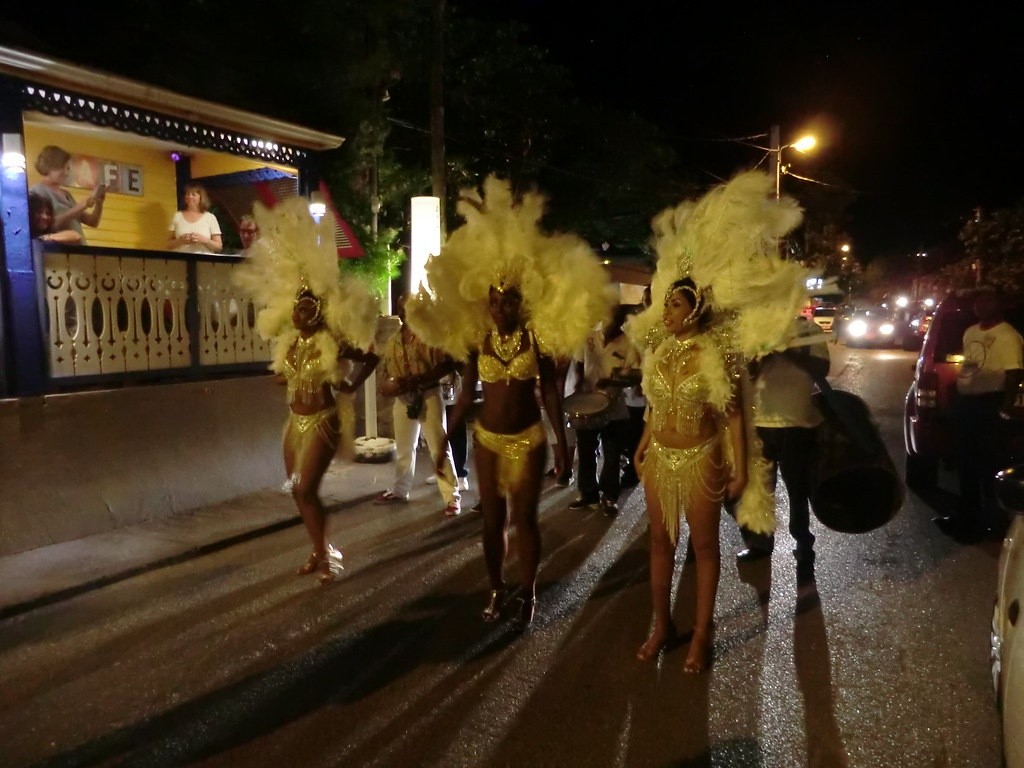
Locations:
[426,370,469,492]
[434,272,571,638]
[240,214,259,249]
[736,314,831,591]
[28,144,110,246]
[569,304,642,518]
[164,180,223,331]
[272,276,384,575]
[931,284,1024,534]
[28,189,84,247]
[633,277,749,675]
[379,290,461,515]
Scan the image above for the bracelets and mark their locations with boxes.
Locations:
[41,233,55,244]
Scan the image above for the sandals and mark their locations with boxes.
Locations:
[513,596,537,630]
[481,584,506,624]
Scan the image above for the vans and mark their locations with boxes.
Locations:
[813,305,838,337]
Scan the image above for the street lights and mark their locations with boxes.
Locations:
[770,125,815,261]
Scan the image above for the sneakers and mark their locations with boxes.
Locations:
[374,491,409,505]
[444,500,461,516]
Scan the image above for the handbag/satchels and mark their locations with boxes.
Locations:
[801,390,908,534]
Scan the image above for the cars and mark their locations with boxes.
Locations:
[984,466,1024,768]
[905,287,1024,545]
[844,309,934,350]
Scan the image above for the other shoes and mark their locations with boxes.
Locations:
[735,548,774,559]
[543,467,558,480]
[297,553,323,575]
[471,503,482,513]
[555,474,575,488]
[794,534,816,555]
[600,495,619,517]
[317,548,344,587]
[459,477,469,491]
[569,495,601,510]
[425,475,438,485]
